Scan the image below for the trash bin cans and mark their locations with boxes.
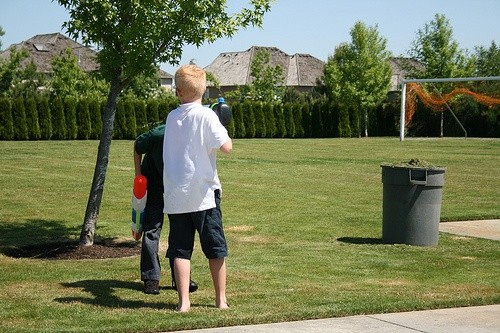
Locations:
[380,164,447,246]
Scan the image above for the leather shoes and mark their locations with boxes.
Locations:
[172,276,197,290]
[144,280,160,294]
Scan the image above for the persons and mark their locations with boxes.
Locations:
[162,63,232,312]
[133,125,198,295]
